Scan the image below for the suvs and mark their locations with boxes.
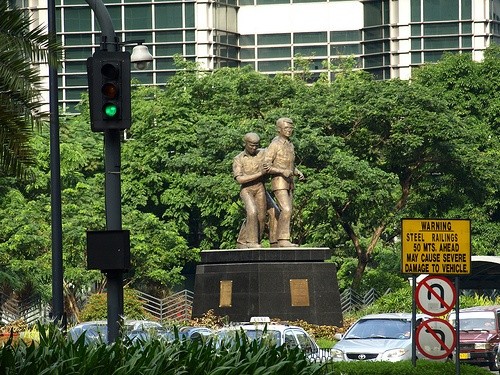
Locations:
[445,306,500,371]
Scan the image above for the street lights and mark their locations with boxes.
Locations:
[98,33,156,342]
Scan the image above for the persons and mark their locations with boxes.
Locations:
[265,116,304,247]
[232,132,272,249]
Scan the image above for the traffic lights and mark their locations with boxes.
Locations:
[99,58,123,124]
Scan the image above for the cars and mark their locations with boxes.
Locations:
[65,319,318,370]
[329,312,454,365]
